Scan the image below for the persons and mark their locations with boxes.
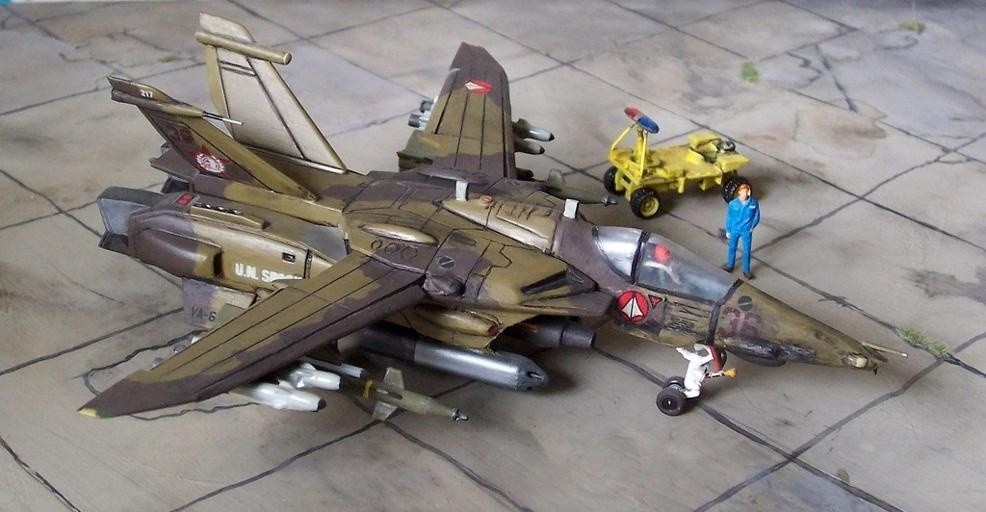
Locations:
[721,185,760,280]
